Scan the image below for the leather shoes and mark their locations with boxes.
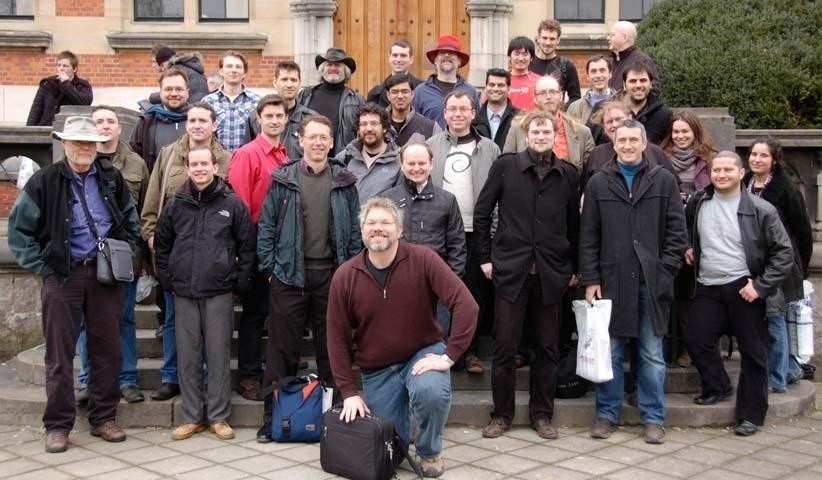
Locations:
[257,424,272,442]
[77,383,178,403]
[592,418,616,437]
[171,421,236,439]
[694,391,731,404]
[91,419,126,442]
[239,379,264,400]
[644,423,667,443]
[735,420,757,435]
[45,431,66,452]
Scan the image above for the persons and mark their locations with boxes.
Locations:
[26,49,94,126]
[326,197,481,479]
[474,111,583,439]
[74,33,502,401]
[682,151,794,436]
[740,136,814,393]
[579,118,685,442]
[153,147,254,440]
[474,18,723,369]
[8,117,142,456]
[256,116,361,443]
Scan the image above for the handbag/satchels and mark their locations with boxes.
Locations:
[270,375,323,443]
[97,237,134,285]
[572,299,614,383]
[322,406,398,480]
[788,295,814,364]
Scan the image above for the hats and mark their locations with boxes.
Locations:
[315,49,356,73]
[156,47,175,65]
[427,35,469,67]
[51,116,111,144]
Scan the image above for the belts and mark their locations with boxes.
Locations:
[72,258,96,266]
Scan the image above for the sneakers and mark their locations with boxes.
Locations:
[466,353,485,373]
[421,455,444,477]
[483,416,558,440]
